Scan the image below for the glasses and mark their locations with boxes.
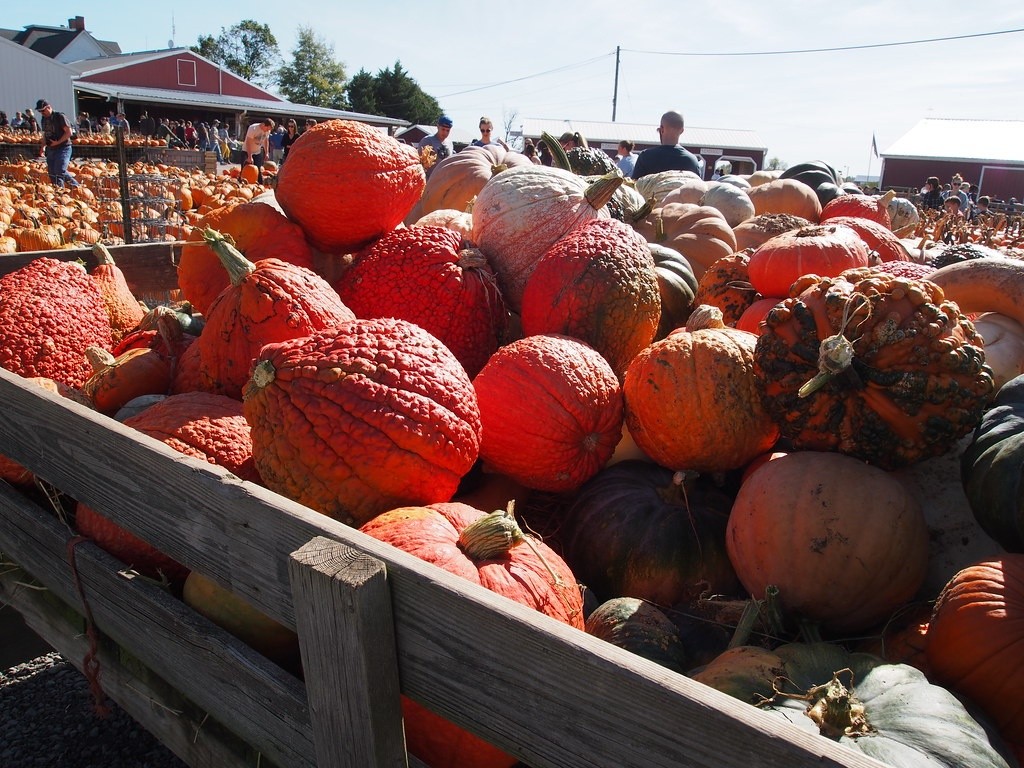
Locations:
[953,183,961,186]
[305,124,310,126]
[925,182,928,185]
[289,125,295,128]
[481,129,491,133]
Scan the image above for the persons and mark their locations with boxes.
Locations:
[76,111,234,165]
[0,109,41,133]
[1008,193,1018,211]
[520,132,639,182]
[238,118,317,185]
[467,116,502,148]
[418,117,454,183]
[631,112,701,181]
[710,168,724,181]
[34,98,79,188]
[910,174,995,226]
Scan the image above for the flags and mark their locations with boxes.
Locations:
[872,132,878,158]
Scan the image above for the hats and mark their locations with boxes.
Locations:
[212,119,219,124]
[34,99,51,110]
[439,116,453,128]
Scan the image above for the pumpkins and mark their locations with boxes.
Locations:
[0,119,1024,768]
